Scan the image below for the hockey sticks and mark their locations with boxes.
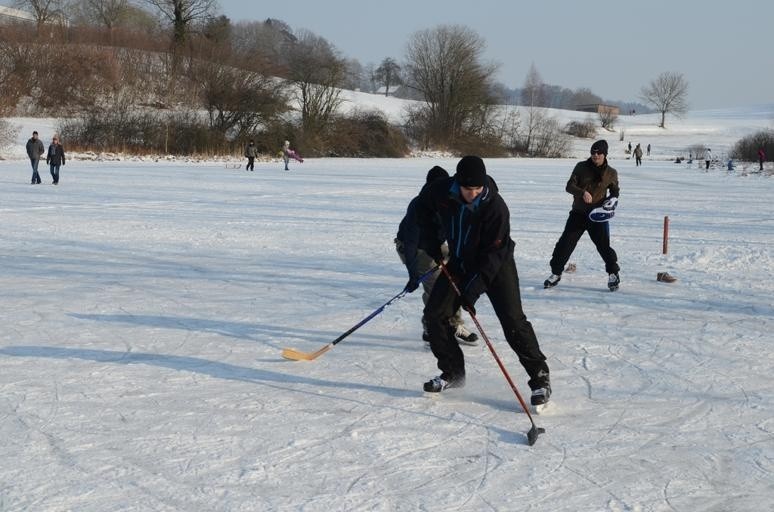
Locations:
[281,268,437,359]
[439,262,538,445]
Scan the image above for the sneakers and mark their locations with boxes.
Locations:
[423,372,467,393]
[424,325,479,344]
[608,271,620,291]
[544,270,562,288]
[657,272,677,282]
[529,370,553,404]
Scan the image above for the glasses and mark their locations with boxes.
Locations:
[591,151,603,154]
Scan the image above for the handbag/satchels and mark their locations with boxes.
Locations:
[279,151,283,155]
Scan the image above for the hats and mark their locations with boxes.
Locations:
[427,155,489,201]
[592,140,608,157]
[52,134,59,140]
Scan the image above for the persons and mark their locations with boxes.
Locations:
[727,160,735,171]
[704,148,712,173]
[686,154,694,165]
[282,140,292,170]
[415,156,552,405]
[757,149,765,170]
[26,131,44,185]
[394,165,478,343]
[627,141,651,167]
[244,139,257,171]
[46,137,65,185]
[632,108,636,117]
[673,157,682,164]
[543,139,620,289]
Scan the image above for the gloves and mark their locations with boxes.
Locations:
[603,196,618,209]
[404,246,446,292]
[244,153,259,158]
[47,159,66,165]
[460,287,484,316]
